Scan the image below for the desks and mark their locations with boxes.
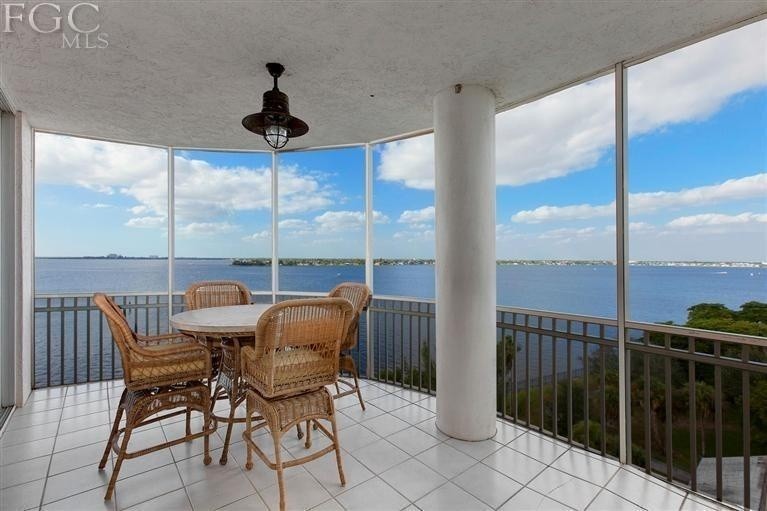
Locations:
[166,304,330,469]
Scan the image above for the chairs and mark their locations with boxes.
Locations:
[238,296,356,511]
[323,281,373,414]
[184,280,255,416]
[89,290,216,503]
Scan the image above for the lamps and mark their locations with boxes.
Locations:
[242,63,309,152]
